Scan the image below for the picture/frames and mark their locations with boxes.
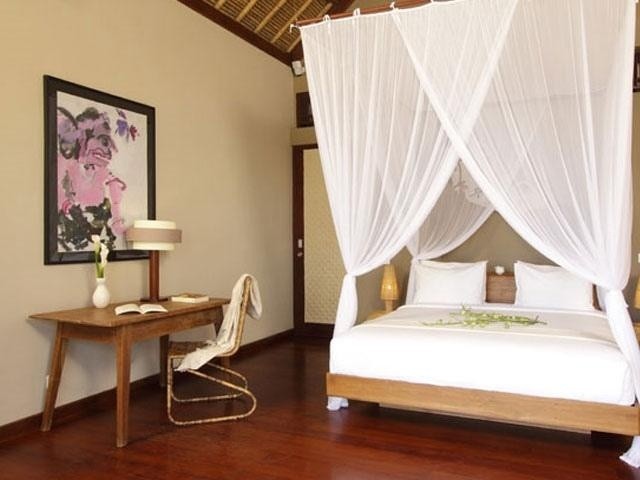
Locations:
[43,73,156,265]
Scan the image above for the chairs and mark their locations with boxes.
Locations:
[166,276,257,426]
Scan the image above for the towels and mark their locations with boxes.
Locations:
[172,273,263,372]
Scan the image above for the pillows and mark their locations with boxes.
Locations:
[412,259,489,306]
[513,260,597,311]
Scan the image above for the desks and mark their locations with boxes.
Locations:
[28,292,230,448]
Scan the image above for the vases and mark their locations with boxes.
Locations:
[92,278,110,309]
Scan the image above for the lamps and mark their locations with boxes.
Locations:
[125,220,182,303]
[381,263,399,312]
[634,275,640,308]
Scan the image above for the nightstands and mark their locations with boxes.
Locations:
[366,310,395,320]
[633,322,640,343]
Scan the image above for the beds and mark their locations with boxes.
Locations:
[326,271,640,438]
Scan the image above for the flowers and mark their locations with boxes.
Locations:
[89,234,110,278]
[419,304,548,331]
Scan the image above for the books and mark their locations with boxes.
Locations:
[115,303,168,316]
[171,293,209,304]
